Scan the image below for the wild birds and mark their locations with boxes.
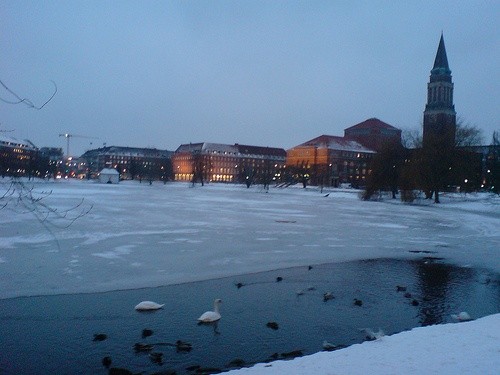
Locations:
[235,282,243,287]
[93,333,108,341]
[142,326,153,337]
[321,340,337,350]
[405,292,412,298]
[353,296,363,305]
[176,340,192,350]
[147,350,164,359]
[308,265,313,270]
[103,356,112,366]
[323,290,333,300]
[396,285,407,291]
[451,312,471,321]
[359,327,384,340]
[185,365,219,375]
[412,300,419,306]
[266,322,279,328]
[134,342,153,351]
[277,277,282,282]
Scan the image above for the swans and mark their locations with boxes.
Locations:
[197,299,223,322]
[135,301,165,310]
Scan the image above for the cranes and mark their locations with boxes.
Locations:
[58,133,95,158]
